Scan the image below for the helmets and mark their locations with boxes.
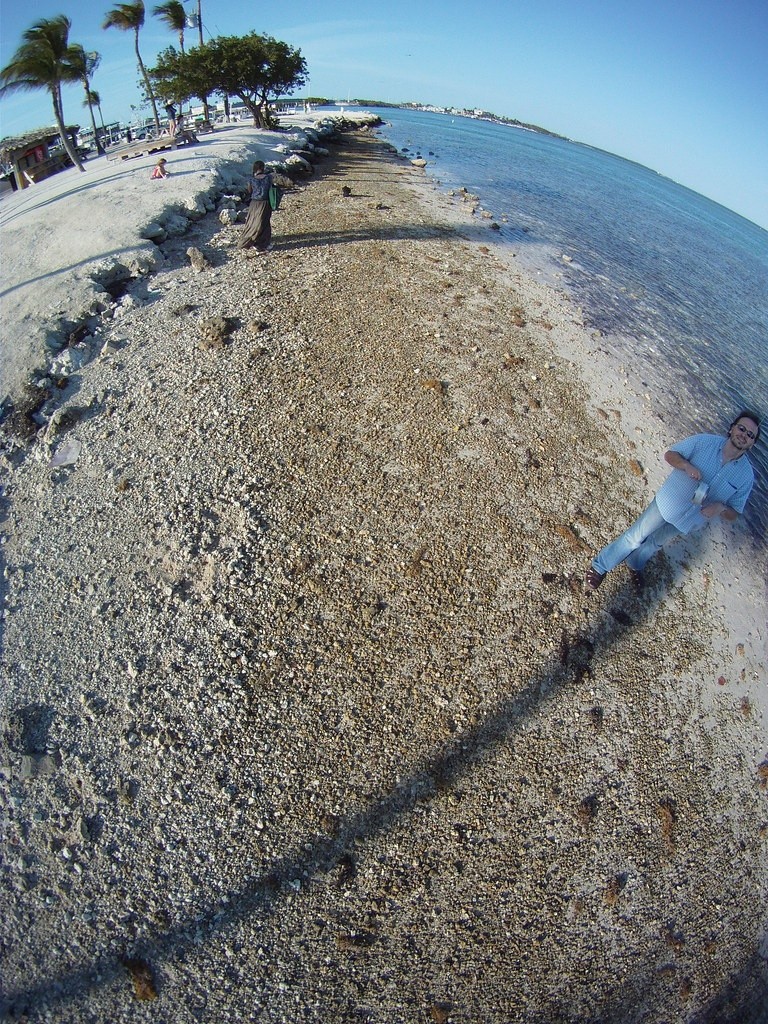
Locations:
[168,100,175,105]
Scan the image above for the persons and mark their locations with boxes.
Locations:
[247,161,273,250]
[585,409,760,587]
[153,158,170,179]
[166,99,177,138]
[176,115,199,146]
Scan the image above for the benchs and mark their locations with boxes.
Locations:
[107,130,196,162]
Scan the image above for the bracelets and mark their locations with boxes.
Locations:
[720,504,728,514]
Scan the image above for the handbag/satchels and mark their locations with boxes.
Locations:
[265,173,283,210]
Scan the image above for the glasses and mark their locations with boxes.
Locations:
[736,423,756,439]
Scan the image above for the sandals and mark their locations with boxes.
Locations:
[586,567,606,589]
[628,567,643,588]
